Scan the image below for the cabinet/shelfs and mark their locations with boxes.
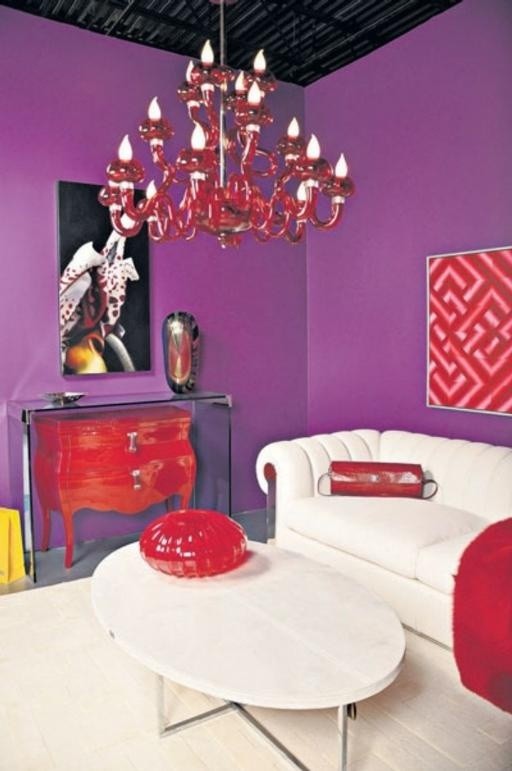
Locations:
[33,406,198,567]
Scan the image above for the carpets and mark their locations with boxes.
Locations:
[0,572,512,771]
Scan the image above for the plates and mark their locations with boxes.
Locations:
[35,391,87,404]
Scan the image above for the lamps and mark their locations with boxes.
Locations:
[97,0,354,250]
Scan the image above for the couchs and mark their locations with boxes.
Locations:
[255,428,511,679]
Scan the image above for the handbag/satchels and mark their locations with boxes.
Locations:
[317,452,438,500]
